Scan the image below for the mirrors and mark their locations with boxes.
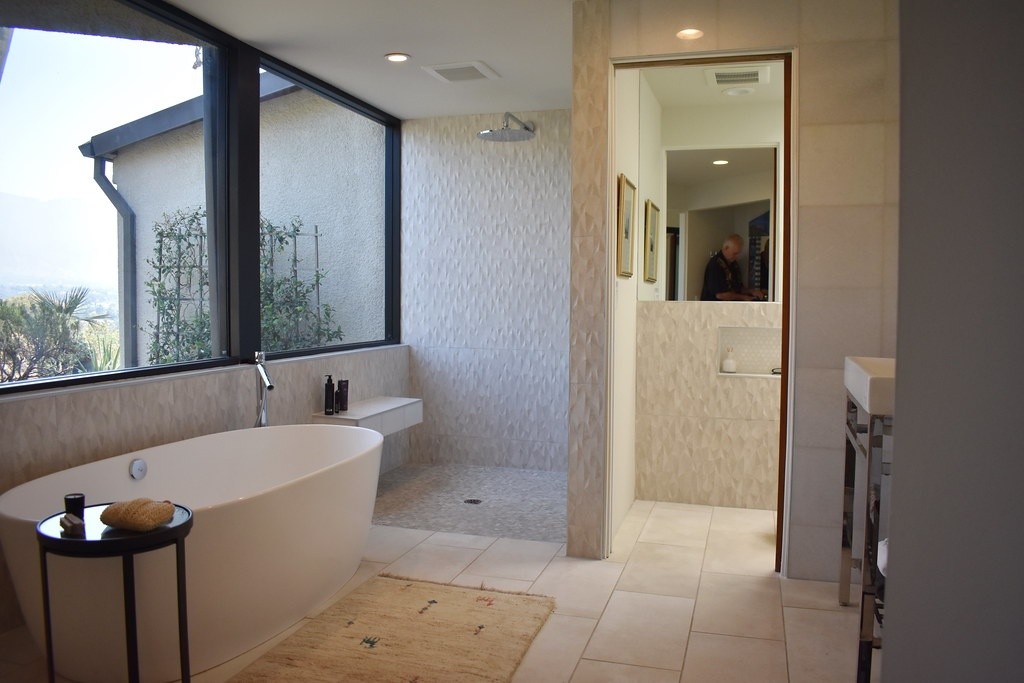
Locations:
[637,59,779,303]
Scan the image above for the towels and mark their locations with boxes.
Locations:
[876,537,887,579]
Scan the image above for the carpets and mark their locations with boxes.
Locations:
[224,576,555,683]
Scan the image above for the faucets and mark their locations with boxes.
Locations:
[254,350,276,428]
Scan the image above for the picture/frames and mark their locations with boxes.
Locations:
[645,199,660,284]
[619,173,636,278]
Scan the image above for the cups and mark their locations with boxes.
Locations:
[64,493,85,521]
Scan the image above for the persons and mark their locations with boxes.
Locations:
[698,232,768,303]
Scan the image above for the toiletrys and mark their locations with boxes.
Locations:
[324,374,349,416]
[721,345,737,373]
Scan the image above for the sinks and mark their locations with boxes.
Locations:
[843,356,896,416]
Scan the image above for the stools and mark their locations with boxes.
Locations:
[36,502,192,683]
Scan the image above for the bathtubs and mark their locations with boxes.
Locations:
[0,424,384,683]
